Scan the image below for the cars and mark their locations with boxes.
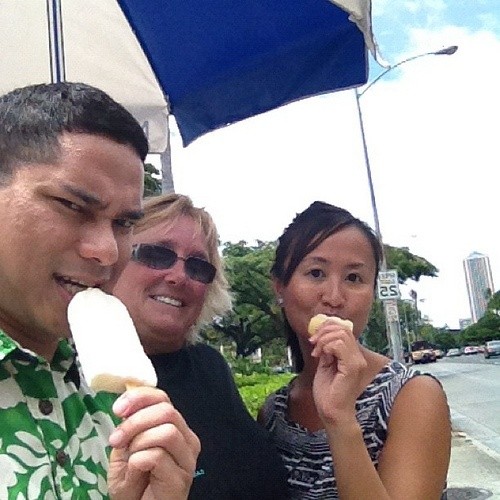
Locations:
[461,345,485,355]
[483,340,500,358]
[433,350,444,358]
[446,348,461,357]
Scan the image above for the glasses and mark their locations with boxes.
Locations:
[130,243,216,284]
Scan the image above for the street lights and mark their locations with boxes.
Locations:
[356,45,457,362]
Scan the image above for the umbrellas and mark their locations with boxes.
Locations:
[0,0,391,154]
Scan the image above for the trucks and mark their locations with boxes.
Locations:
[411,341,436,364]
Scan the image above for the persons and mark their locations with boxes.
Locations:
[111,193,288,500]
[0,83,201,500]
[256,200,452,500]
